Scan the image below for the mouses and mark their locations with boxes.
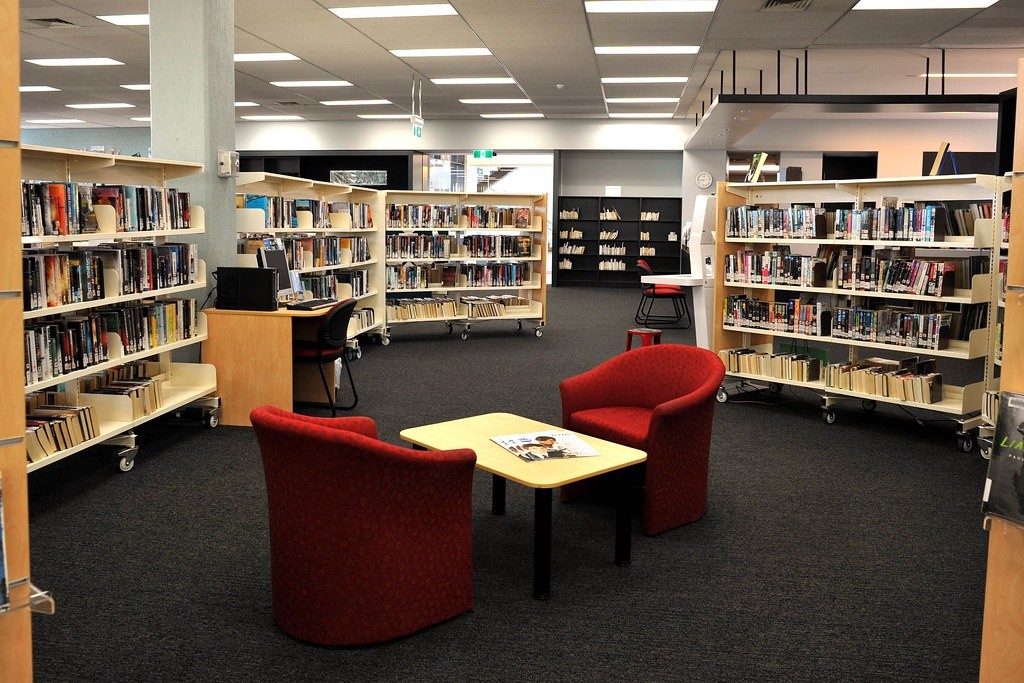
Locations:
[322,297,333,300]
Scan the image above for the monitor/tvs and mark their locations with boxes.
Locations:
[255,245,293,308]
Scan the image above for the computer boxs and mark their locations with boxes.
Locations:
[215,266,278,311]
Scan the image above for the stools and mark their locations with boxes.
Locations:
[625,327,661,352]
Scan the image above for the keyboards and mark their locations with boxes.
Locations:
[287,299,338,311]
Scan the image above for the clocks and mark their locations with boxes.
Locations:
[695,171,712,189]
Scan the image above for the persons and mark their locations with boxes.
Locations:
[509,436,576,463]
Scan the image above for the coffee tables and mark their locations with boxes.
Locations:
[398,411,649,602]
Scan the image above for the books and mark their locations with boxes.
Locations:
[722,333,987,407]
[21,179,191,235]
[721,282,1004,360]
[720,241,1007,307]
[490,429,602,464]
[344,305,377,339]
[293,270,370,301]
[24,295,200,385]
[242,232,372,269]
[724,199,1010,245]
[23,358,170,464]
[23,241,198,310]
[234,192,375,231]
[385,202,537,318]
[559,210,678,270]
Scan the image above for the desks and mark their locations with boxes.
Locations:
[198,304,335,429]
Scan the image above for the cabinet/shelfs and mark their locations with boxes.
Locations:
[17,142,219,476]
[236,171,549,360]
[555,195,683,285]
[714,170,1013,459]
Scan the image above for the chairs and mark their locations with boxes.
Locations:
[292,297,359,418]
[634,259,692,330]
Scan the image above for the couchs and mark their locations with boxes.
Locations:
[250,406,477,646]
[557,344,725,536]
